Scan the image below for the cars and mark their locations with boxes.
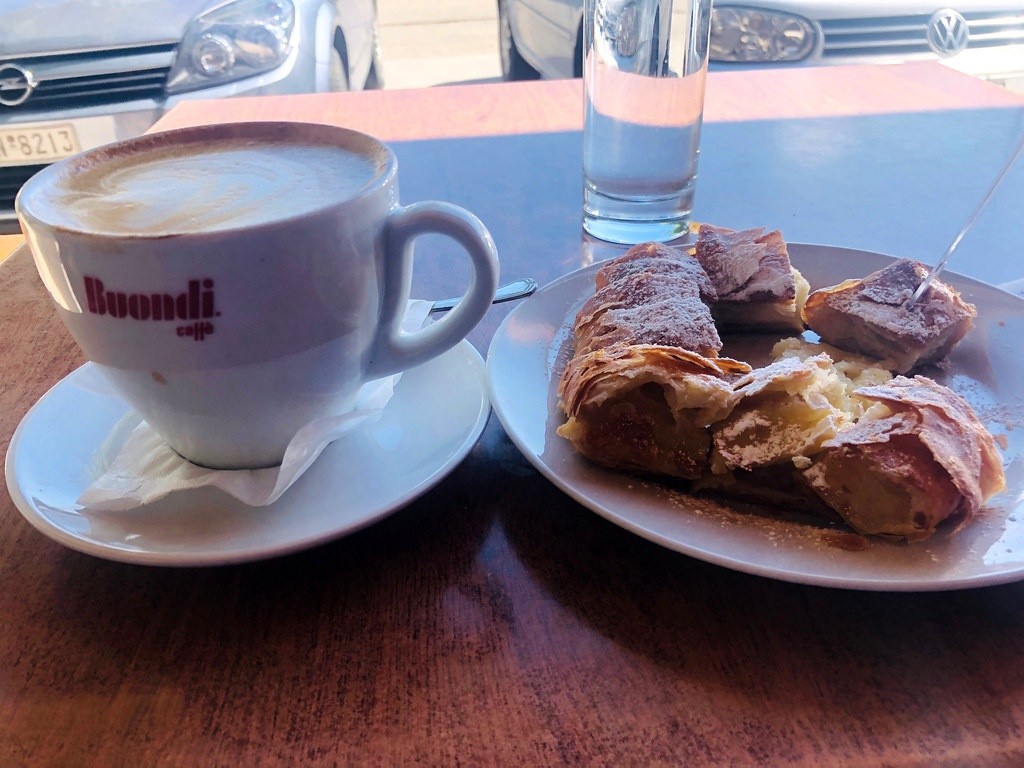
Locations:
[0,0,386,221]
[497,0,1024,82]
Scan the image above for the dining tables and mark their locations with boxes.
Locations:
[0,60,1024,768]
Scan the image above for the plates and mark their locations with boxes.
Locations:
[4,316,491,563]
[489,242,1023,592]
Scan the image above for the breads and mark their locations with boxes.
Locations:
[553,223,1006,540]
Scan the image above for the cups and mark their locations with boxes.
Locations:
[581,1,713,248]
[14,121,501,468]
